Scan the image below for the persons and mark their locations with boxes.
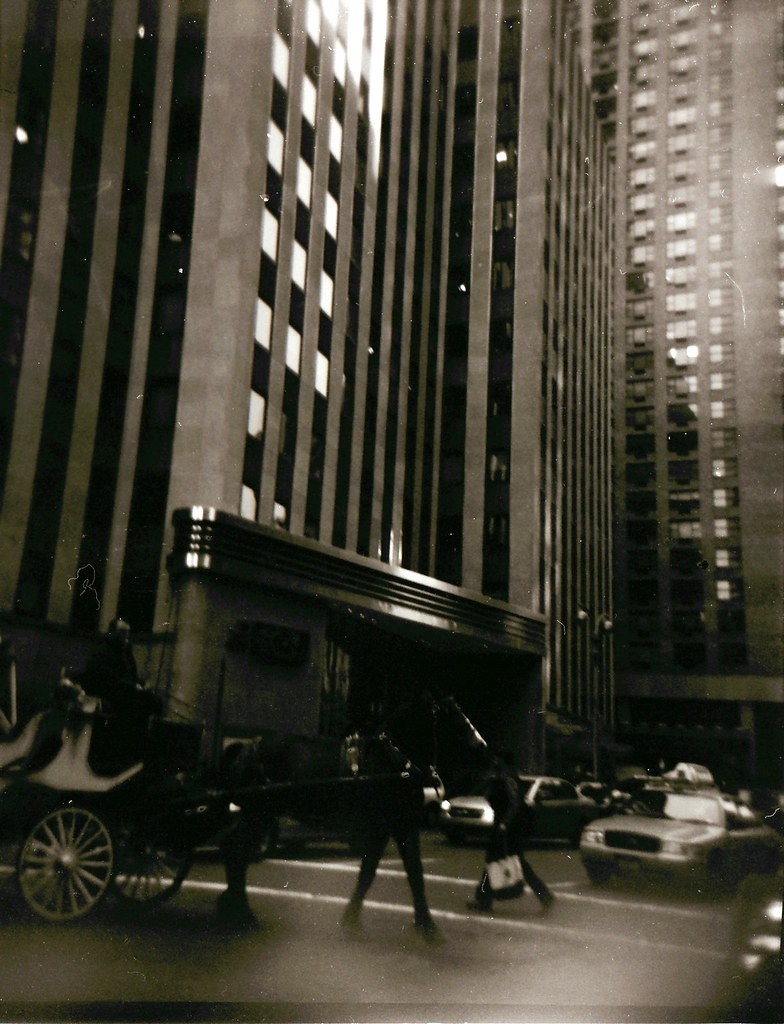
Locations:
[467,754,557,917]
[80,617,151,738]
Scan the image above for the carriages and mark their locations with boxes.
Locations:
[0,600,489,963]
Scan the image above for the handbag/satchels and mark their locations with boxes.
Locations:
[484,855,526,893]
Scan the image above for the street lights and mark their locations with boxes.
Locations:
[590,604,614,777]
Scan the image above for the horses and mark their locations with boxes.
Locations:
[201,682,490,950]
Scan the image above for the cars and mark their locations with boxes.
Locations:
[577,780,784,898]
[438,774,596,849]
